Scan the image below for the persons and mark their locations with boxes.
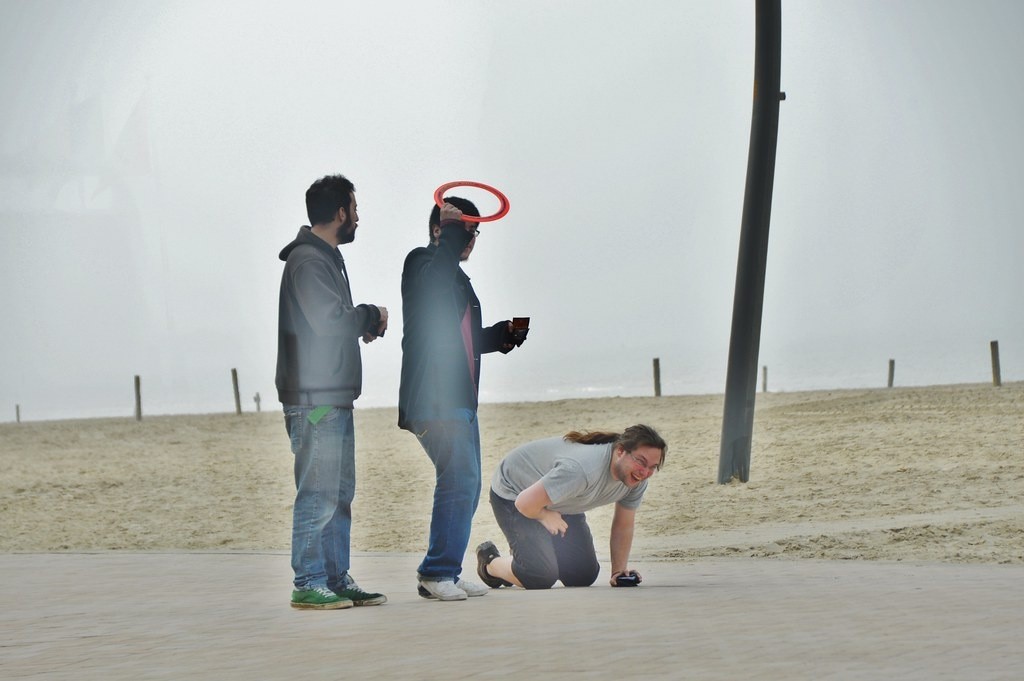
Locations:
[275,174,389,611]
[397,196,529,601]
[476,424,668,590]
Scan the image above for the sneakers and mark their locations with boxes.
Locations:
[418,579,488,599]
[476,541,503,588]
[416,571,467,600]
[290,582,353,609]
[501,578,513,587]
[327,573,386,606]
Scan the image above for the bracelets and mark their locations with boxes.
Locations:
[611,571,620,579]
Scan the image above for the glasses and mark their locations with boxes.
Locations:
[627,449,657,474]
[473,229,480,238]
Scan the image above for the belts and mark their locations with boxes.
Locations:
[307,406,334,425]
